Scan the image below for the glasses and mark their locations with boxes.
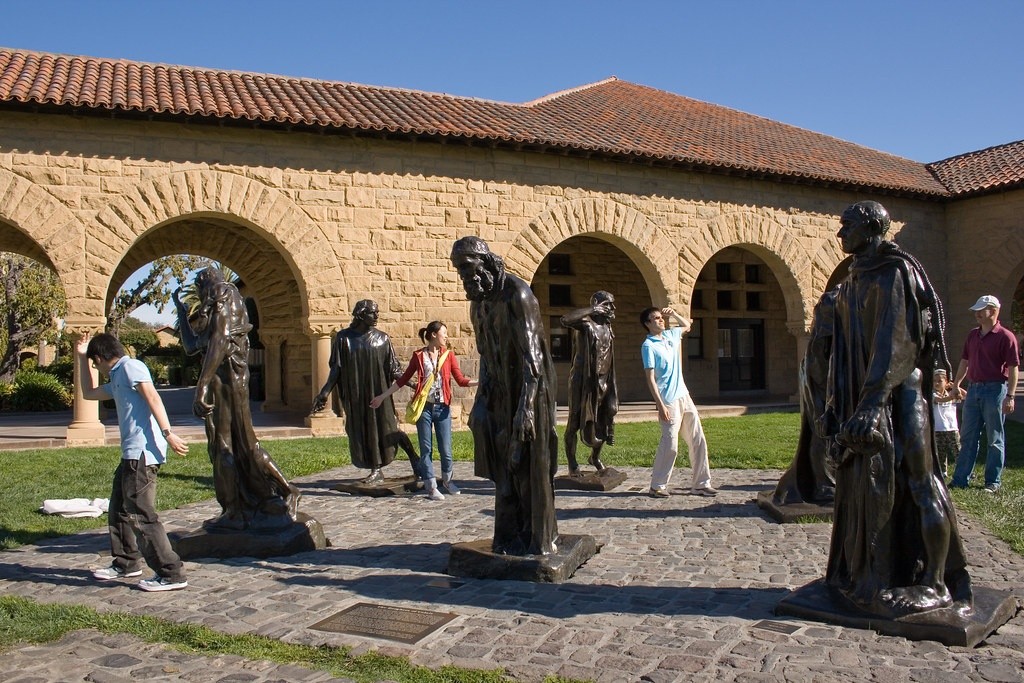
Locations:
[649,315,664,321]
[91,360,97,368]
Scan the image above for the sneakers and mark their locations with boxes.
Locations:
[692,486,720,496]
[650,487,671,498]
[443,481,460,494]
[94,564,143,579]
[428,488,445,500]
[139,575,188,592]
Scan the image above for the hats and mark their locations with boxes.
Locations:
[968,295,1001,311]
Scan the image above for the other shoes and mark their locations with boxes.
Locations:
[942,471,948,477]
[984,484,997,492]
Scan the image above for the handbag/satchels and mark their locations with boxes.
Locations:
[404,391,426,424]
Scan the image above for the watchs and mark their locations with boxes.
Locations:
[162,429,172,439]
[1006,394,1015,398]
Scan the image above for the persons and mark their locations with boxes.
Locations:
[818,199,975,610]
[451,233,564,556]
[775,313,844,506]
[309,300,432,490]
[559,290,620,475]
[640,305,719,498]
[927,367,967,476]
[168,266,304,532]
[75,330,189,591]
[943,295,1020,495]
[366,321,480,501]
